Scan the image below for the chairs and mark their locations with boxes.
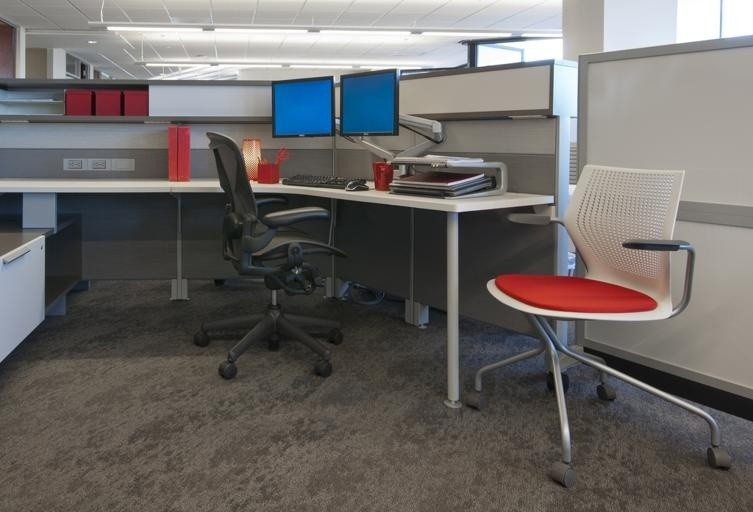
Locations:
[464,162,731,489]
[193,129,343,381]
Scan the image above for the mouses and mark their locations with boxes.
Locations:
[345,181,367,191]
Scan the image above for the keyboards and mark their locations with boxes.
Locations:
[282,174,366,188]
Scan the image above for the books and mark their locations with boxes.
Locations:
[394,155,483,164]
[392,171,483,186]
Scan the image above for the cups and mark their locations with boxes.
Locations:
[257,163,281,184]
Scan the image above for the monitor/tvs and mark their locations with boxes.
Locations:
[271,76,335,137]
[341,67,400,136]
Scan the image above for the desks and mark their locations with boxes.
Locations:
[0,178,553,411]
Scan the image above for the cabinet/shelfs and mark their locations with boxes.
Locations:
[0,78,149,121]
[0,212,84,362]
